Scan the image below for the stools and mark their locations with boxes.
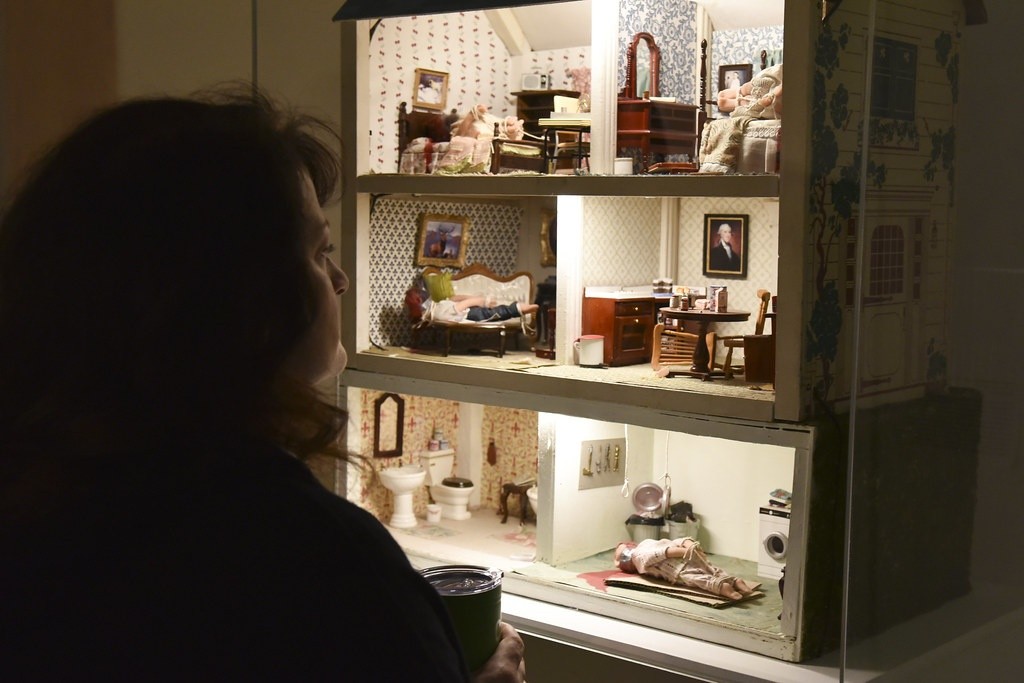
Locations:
[499,482,533,524]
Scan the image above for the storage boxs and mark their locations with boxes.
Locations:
[709,285,727,310]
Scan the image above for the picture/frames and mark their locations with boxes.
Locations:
[538,205,556,267]
[411,211,470,268]
[413,66,450,111]
[719,64,754,112]
[702,213,749,278]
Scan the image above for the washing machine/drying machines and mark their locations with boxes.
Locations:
[755,494,793,578]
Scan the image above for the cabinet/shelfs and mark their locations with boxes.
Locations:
[616,99,698,174]
[581,296,657,369]
[330,0,966,422]
[509,88,582,170]
[329,367,981,661]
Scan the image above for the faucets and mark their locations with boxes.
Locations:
[399,458,403,467]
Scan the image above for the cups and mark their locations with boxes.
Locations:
[420,565,503,674]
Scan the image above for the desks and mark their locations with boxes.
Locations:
[534,282,555,344]
[537,117,591,173]
[658,307,752,382]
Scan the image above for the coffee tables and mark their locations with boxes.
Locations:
[441,324,506,357]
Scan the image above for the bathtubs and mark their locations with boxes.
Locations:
[526,486,538,514]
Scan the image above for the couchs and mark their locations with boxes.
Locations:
[404,263,538,348]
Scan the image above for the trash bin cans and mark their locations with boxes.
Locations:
[613,158,633,175]
[426,504,441,522]
[633,483,664,544]
[664,486,700,541]
[574,335,609,368]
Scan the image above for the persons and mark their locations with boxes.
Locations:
[710,224,741,271]
[614,538,753,600]
[420,270,539,322]
[1,100,525,681]
[730,72,740,89]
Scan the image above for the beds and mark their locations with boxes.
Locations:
[696,36,782,176]
[397,101,551,175]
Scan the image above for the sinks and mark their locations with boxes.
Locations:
[379,465,427,493]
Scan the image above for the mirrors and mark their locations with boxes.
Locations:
[372,392,404,458]
[624,30,662,99]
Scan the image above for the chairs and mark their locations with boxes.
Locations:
[651,322,745,375]
[723,289,771,377]
[550,95,590,174]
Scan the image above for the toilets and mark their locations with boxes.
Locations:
[419,448,473,519]
